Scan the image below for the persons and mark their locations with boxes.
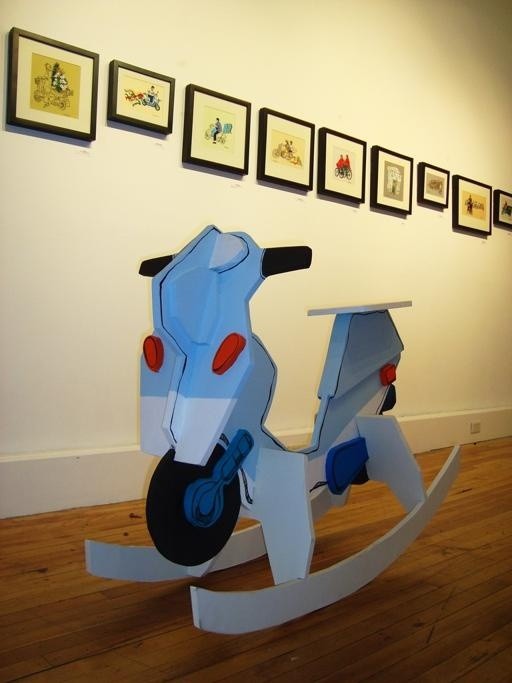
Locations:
[148,84,156,101]
[290,141,296,157]
[211,116,222,143]
[336,154,344,168]
[40,62,51,108]
[52,62,68,91]
[342,154,351,176]
[283,139,292,160]
